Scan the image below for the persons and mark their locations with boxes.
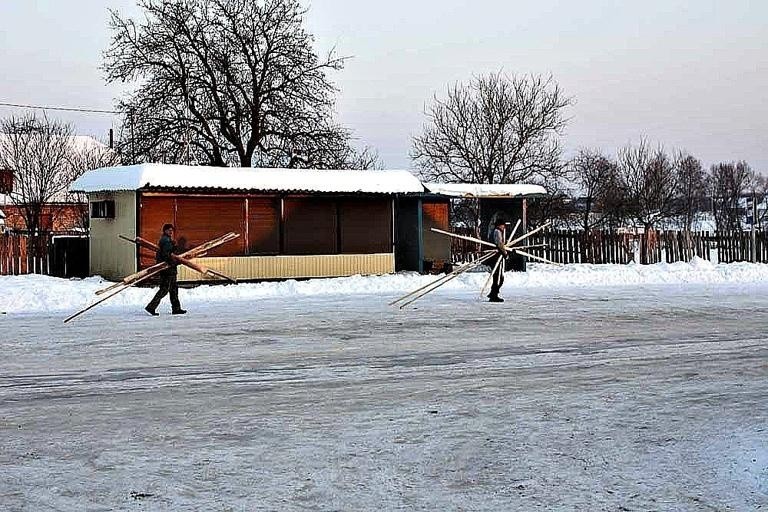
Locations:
[145,223,186,317]
[485,219,508,303]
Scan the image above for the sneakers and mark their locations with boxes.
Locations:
[144,306,187,316]
[487,291,505,302]
